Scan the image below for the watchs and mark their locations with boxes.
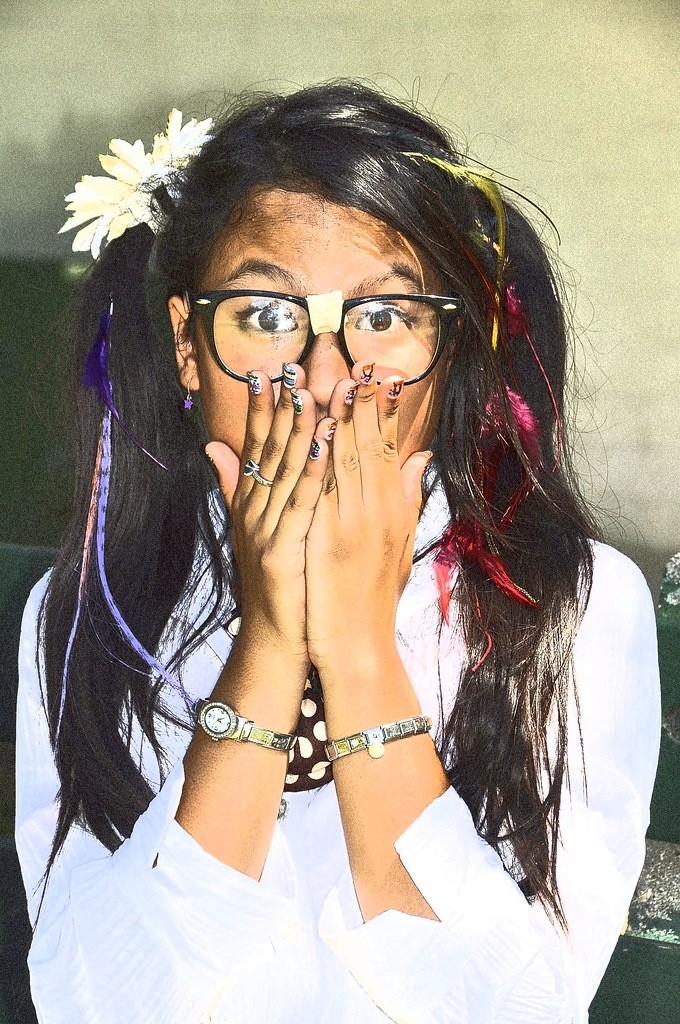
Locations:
[191,695,298,750]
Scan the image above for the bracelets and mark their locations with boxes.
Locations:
[324,711,432,764]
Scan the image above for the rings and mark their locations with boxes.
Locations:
[242,456,275,488]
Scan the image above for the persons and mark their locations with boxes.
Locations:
[15,77,662,1024]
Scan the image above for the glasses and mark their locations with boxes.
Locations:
[180,289,463,386]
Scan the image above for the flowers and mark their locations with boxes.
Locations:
[52,107,213,257]
